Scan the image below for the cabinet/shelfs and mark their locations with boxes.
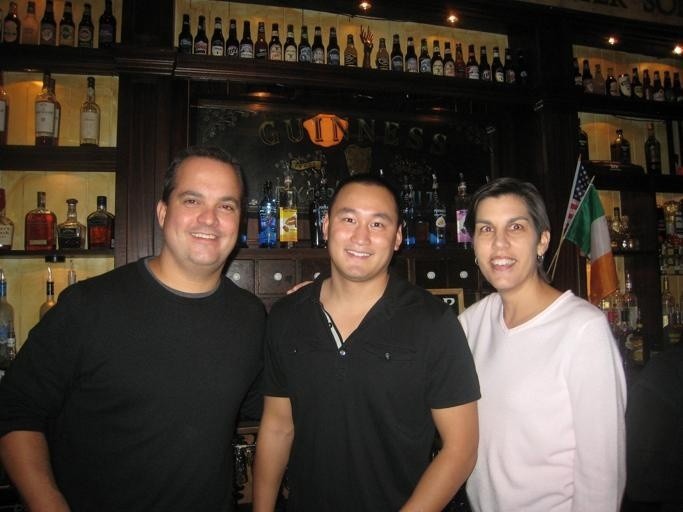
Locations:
[544,0,681,363]
[156,0,530,297]
[0,1,116,374]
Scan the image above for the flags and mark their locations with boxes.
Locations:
[564,183,620,303]
[563,162,590,236]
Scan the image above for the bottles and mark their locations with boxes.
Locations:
[466,44,479,79]
[77,2,94,48]
[582,59,593,95]
[644,122,661,176]
[87,196,114,252]
[431,39,443,77]
[39,0,56,45]
[479,45,490,80]
[593,64,605,96]
[419,38,431,73]
[606,68,619,98]
[443,41,454,77]
[375,38,389,70]
[178,14,192,54]
[454,42,466,79]
[576,117,589,161]
[490,46,505,88]
[310,169,329,249]
[672,72,682,103]
[210,17,224,56]
[284,23,297,62]
[253,21,268,60]
[663,71,672,103]
[297,25,311,63]
[344,34,357,67]
[257,179,276,248]
[19,0,38,44]
[642,69,652,101]
[225,19,239,57]
[608,128,630,163]
[598,268,646,368]
[39,265,57,320]
[279,174,298,249]
[573,56,583,90]
[455,173,473,251]
[398,175,415,250]
[609,206,639,253]
[405,37,417,73]
[327,27,340,65]
[311,26,325,64]
[652,70,664,101]
[517,55,528,88]
[0,268,17,372]
[0,87,9,145]
[661,275,682,328]
[390,34,403,71]
[503,48,517,89]
[0,188,15,254]
[58,1,75,47]
[23,191,57,253]
[268,23,282,61]
[79,76,100,146]
[427,173,447,250]
[630,67,643,99]
[34,72,61,148]
[97,0,116,47]
[2,1,21,43]
[56,198,86,251]
[193,15,208,54]
[239,20,253,58]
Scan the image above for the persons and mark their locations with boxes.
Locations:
[249,173,481,512]
[457,177,628,512]
[0,145,267,512]
